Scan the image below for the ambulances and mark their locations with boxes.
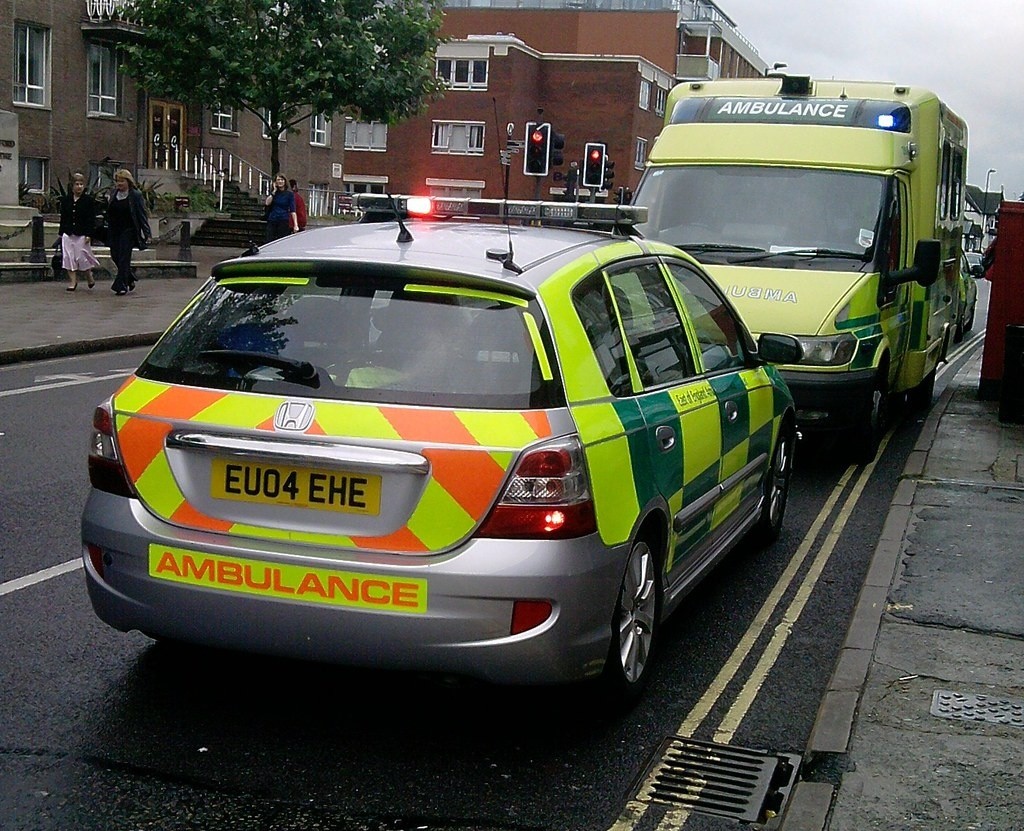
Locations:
[79,194,804,703]
[611,75,969,438]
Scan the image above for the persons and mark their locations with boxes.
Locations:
[58,173,96,294]
[105,169,152,292]
[264,171,299,245]
[288,179,306,233]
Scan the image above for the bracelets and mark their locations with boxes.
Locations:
[270,193,274,197]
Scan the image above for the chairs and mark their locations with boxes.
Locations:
[277,295,361,369]
[376,288,459,362]
[458,305,537,388]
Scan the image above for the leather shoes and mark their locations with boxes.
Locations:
[88,275,95,289]
[66,282,77,291]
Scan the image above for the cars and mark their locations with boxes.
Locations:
[954,251,984,344]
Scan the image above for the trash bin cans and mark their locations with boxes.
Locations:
[998,324,1024,425]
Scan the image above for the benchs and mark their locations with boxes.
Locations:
[337,193,367,217]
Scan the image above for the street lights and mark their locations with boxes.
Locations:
[979,169,996,251]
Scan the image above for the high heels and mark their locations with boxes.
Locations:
[128,274,138,291]
[117,285,128,295]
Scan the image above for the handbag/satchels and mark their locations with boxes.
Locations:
[51,240,65,272]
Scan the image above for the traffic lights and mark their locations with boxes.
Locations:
[550,123,565,168]
[598,155,615,193]
[583,142,607,188]
[522,121,551,177]
[613,186,623,205]
